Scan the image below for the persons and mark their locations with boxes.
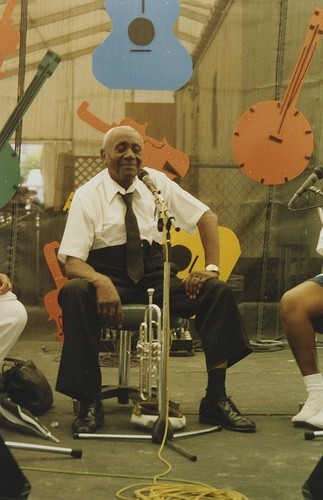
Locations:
[279,207,323,427]
[0,273,28,369]
[56,125,257,432]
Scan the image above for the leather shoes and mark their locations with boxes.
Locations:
[199,395,256,431]
[72,399,105,434]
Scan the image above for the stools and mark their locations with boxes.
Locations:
[73,304,182,415]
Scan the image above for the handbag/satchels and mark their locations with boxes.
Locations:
[0,357,53,416]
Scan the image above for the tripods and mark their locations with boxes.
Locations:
[73,185,223,462]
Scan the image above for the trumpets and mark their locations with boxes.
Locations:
[135,287,163,413]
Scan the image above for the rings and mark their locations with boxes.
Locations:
[194,278,200,280]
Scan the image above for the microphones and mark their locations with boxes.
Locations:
[136,168,157,194]
[288,165,323,207]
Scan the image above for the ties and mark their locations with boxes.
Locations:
[122,192,144,283]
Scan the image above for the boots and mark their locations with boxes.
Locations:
[291,373,323,430]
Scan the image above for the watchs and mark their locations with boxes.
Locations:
[206,264,220,274]
